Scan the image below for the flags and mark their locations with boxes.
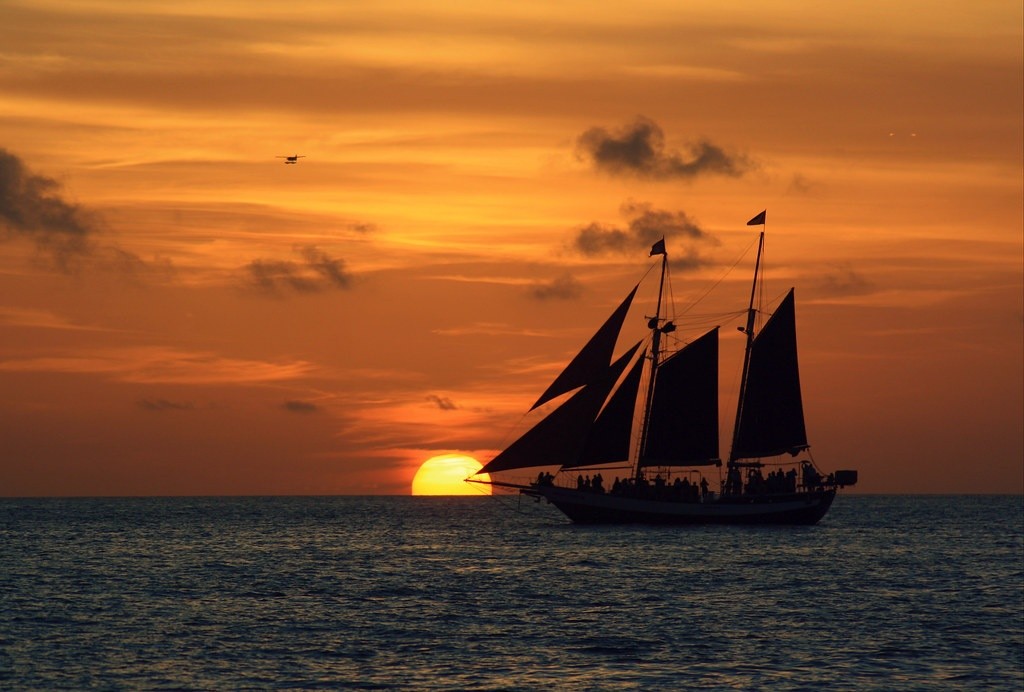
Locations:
[649,240,664,256]
[746,211,766,225]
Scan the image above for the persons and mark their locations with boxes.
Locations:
[538,461,821,492]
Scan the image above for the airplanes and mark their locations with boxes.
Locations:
[274,153,306,165]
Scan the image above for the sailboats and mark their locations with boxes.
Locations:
[463,208,858,528]
